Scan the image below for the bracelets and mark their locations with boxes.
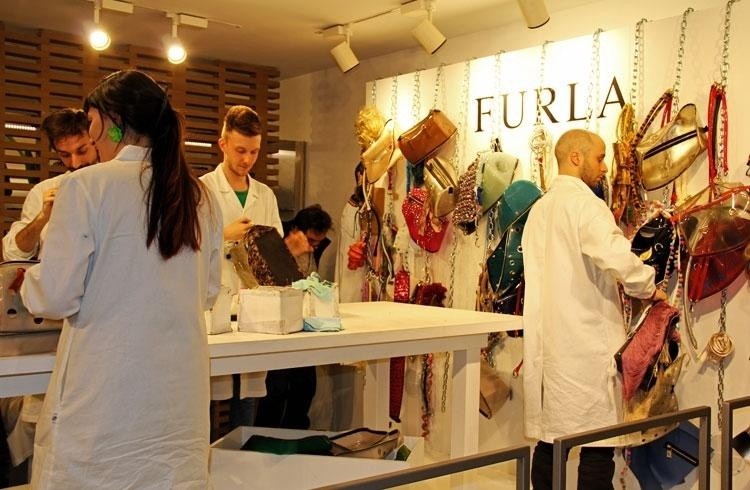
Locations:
[650,289,658,301]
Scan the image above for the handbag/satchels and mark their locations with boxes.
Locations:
[412,281,447,306]
[229,225,306,286]
[630,208,699,286]
[622,421,714,490]
[636,103,708,192]
[361,119,403,185]
[292,272,342,332]
[486,181,544,291]
[398,110,458,167]
[402,187,450,252]
[329,427,403,459]
[453,159,481,236]
[0,261,63,336]
[479,152,519,214]
[423,157,460,218]
[671,181,750,304]
[615,298,680,393]
[623,354,685,440]
[479,357,509,419]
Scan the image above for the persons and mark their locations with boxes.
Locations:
[16,67,227,490]
[520,127,669,490]
[254,202,333,429]
[2,106,100,482]
[198,102,285,428]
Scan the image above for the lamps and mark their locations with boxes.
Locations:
[330,1,550,74]
[83,1,189,69]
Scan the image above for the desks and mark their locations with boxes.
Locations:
[1,301,525,461]
